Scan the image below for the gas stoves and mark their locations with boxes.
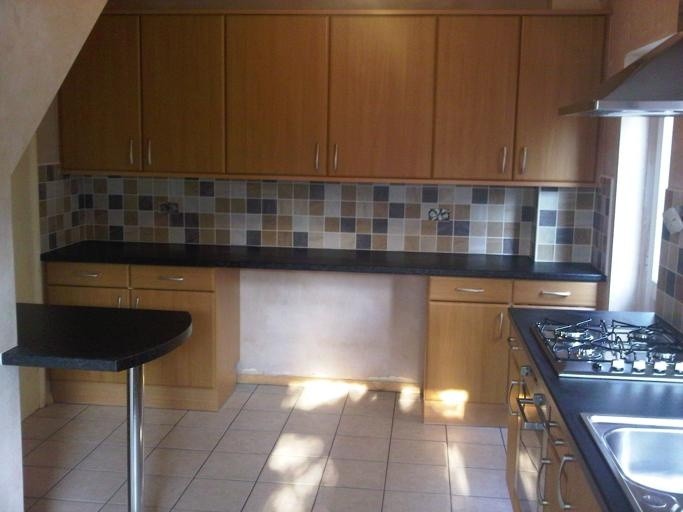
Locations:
[531,315,682,381]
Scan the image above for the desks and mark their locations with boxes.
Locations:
[2,303,191,512]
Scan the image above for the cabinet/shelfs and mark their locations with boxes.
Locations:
[45,262,240,412]
[432,9,612,187]
[505,321,609,512]
[225,10,432,184]
[423,276,597,427]
[59,10,224,179]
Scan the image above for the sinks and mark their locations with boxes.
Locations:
[579,411,683,512]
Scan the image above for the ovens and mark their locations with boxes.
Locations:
[512,381,551,512]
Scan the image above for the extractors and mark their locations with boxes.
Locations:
[557,34,683,120]
